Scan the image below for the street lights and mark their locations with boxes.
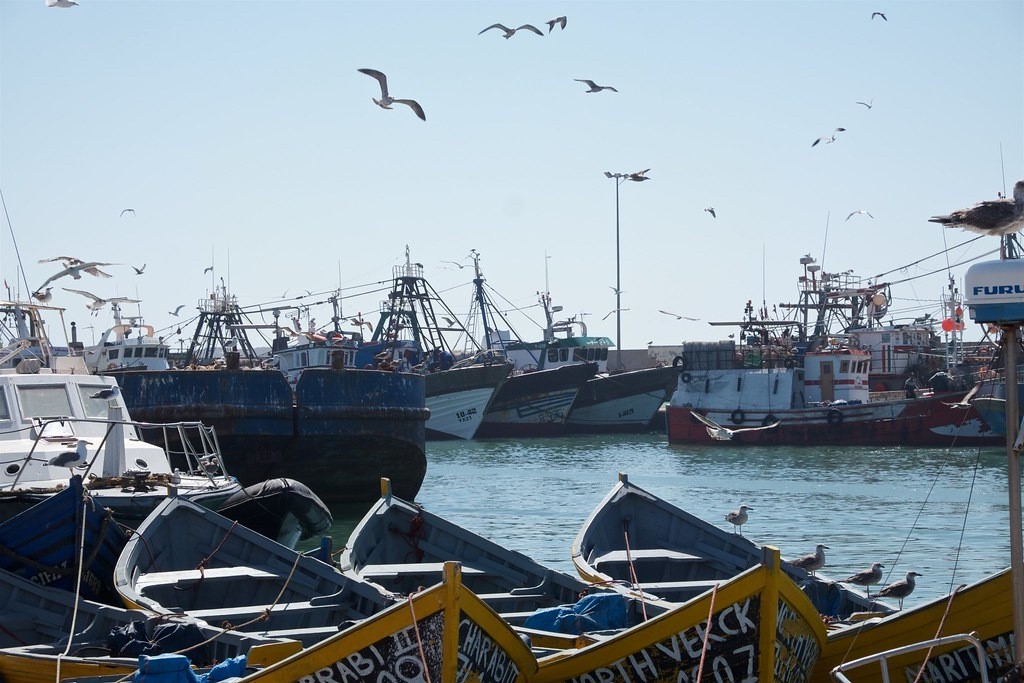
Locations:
[604,170,629,370]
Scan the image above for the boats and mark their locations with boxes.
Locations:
[113,481,540,683]
[0,475,132,612]
[570,468,1024,682]
[0,187,1024,553]
[338,475,830,683]
[0,569,303,683]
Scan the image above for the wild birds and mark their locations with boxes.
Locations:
[31,256,146,318]
[42,439,93,478]
[629,168,651,181]
[90,385,123,407]
[272,326,317,342]
[168,305,185,317]
[939,382,982,410]
[609,287,626,295]
[602,308,631,320]
[545,16,567,34]
[811,128,845,146]
[835,562,922,611]
[928,180,1024,260]
[724,506,754,535]
[686,407,782,440]
[432,259,483,273]
[120,209,136,217]
[704,207,716,218]
[478,23,544,40]
[347,316,373,333]
[871,12,888,21]
[48,0,79,8]
[856,98,876,109]
[573,78,618,93]
[357,68,425,120]
[785,545,830,580]
[845,210,875,222]
[658,309,700,321]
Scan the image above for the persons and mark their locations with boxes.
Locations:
[783,327,790,338]
[904,372,919,399]
[928,372,956,394]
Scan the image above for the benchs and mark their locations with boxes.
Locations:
[246,625,340,648]
[631,579,729,602]
[359,563,502,582]
[498,611,533,626]
[593,547,712,582]
[0,637,106,657]
[183,601,354,631]
[135,565,280,611]
[475,593,555,604]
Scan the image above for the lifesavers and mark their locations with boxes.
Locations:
[762,415,779,433]
[682,372,692,384]
[731,410,745,424]
[784,356,793,369]
[828,410,843,426]
[672,355,687,372]
